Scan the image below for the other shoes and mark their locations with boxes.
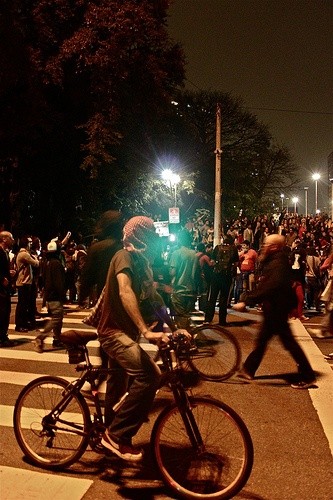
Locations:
[100,425,142,462]
[32,337,42,353]
[51,339,66,349]
[234,368,253,382]
[14,326,27,333]
[324,352,333,362]
[290,374,316,389]
[0,337,17,347]
[307,328,324,338]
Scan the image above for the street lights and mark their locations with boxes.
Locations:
[280,194,284,210]
[292,197,298,214]
[312,173,320,213]
[160,168,182,246]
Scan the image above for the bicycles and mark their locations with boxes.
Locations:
[13,311,254,500]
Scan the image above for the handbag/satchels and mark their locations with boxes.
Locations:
[319,279,332,302]
[81,283,105,328]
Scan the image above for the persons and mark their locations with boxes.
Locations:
[13,236,39,332]
[30,236,42,317]
[83,215,192,462]
[2,237,87,303]
[31,236,70,352]
[77,209,130,393]
[0,231,19,348]
[148,205,333,342]
[230,233,318,389]
